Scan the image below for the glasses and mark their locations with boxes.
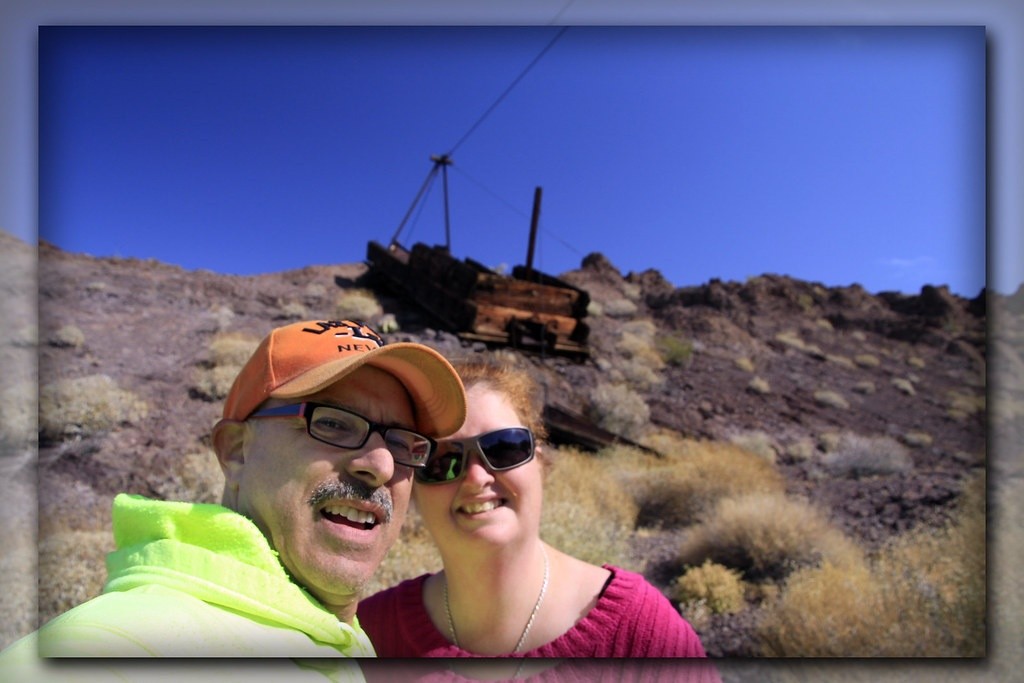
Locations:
[254,403,438,470]
[412,425,536,484]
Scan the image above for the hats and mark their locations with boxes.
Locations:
[224,316,465,439]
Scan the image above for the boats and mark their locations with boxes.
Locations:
[363,153,590,363]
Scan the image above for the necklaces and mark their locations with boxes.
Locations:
[443,544,549,653]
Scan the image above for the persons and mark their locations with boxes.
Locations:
[356,350,709,657]
[40,318,466,658]
